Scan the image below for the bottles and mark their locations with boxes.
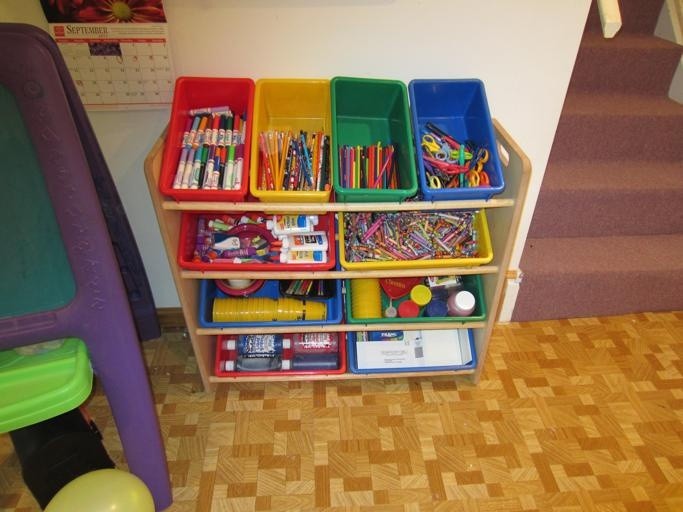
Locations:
[447,288,476,316]
[225,334,282,355]
[282,334,339,352]
[282,352,338,371]
[266,215,329,265]
[224,355,282,372]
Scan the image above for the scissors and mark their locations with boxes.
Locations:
[421,134,492,189]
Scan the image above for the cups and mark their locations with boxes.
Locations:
[212,297,327,322]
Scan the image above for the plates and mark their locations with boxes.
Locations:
[350,278,381,319]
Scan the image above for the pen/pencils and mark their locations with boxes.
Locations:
[284,279,324,295]
[173,106,403,190]
[425,121,469,152]
[343,210,480,263]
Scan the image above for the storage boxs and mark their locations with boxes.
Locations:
[348,331,478,373]
[197,216,343,327]
[346,277,488,324]
[159,76,254,202]
[338,208,494,270]
[214,330,346,379]
[177,210,336,272]
[329,75,420,202]
[408,77,505,202]
[248,78,332,214]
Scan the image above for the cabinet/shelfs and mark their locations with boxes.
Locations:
[144,119,532,394]
